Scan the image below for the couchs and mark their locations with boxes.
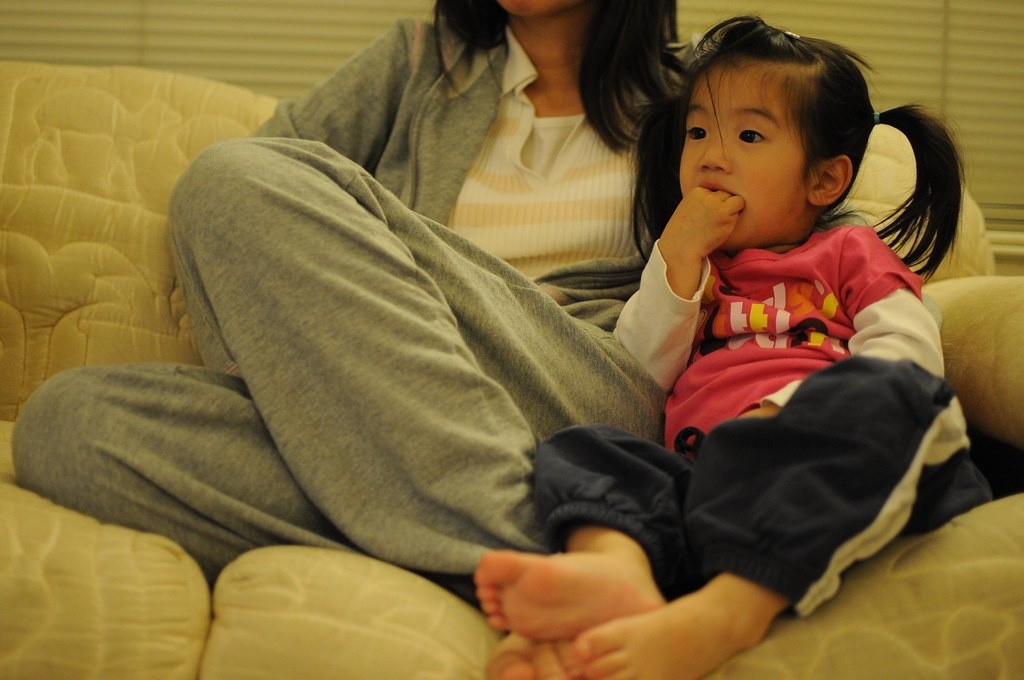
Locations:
[0,58,1024,680]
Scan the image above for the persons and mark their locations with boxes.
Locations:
[476,14,993,680]
[12,0,864,680]
